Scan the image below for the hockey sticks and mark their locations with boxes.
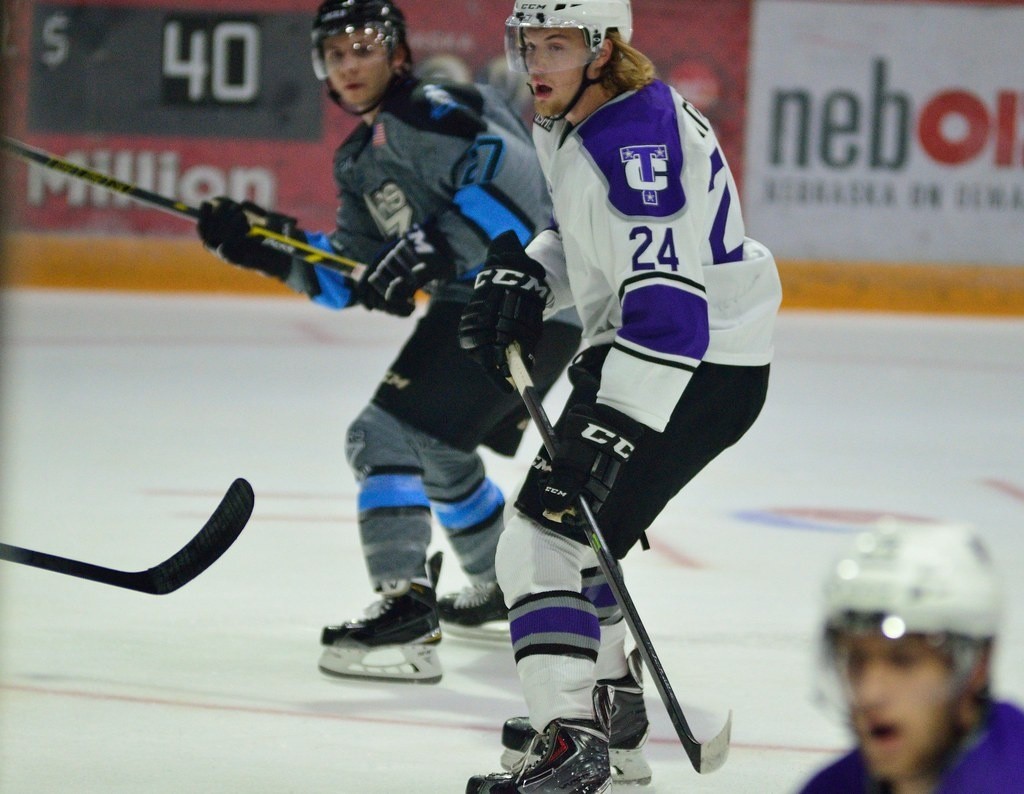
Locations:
[1,477,259,596]
[1,130,367,279]
[507,343,734,776]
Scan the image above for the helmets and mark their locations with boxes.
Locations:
[310,0,407,54]
[824,515,997,641]
[506,0,633,51]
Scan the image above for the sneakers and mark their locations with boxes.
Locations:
[320,550,447,684]
[467,686,618,794]
[438,576,515,646]
[499,640,650,786]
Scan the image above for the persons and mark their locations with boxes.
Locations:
[794,520,1024,794]
[459,0,781,794]
[196,0,583,682]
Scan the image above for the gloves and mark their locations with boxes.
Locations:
[342,232,451,317]
[197,193,296,283]
[453,252,554,379]
[529,419,637,530]
